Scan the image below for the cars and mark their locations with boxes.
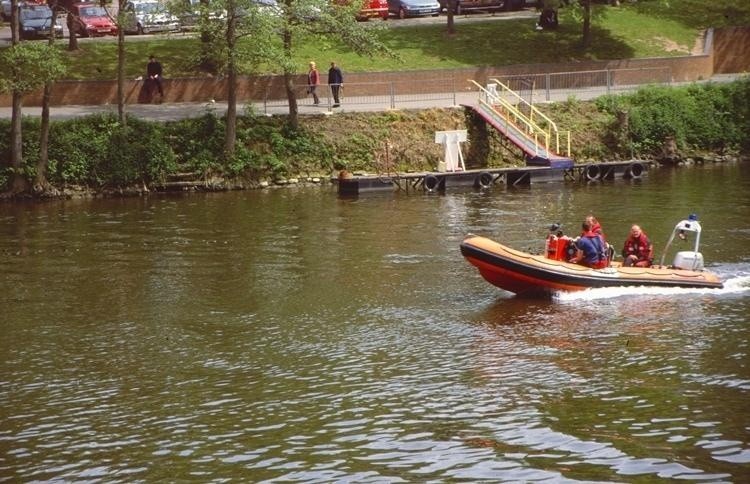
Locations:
[18,6,62,40]
[120,0,284,33]
[387,1,441,19]
[0,0,46,21]
[328,0,390,22]
[67,2,118,36]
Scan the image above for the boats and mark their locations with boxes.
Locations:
[460,233,723,299]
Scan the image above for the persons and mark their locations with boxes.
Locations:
[581,215,606,242]
[327,61,345,107]
[305,60,322,106]
[144,55,164,104]
[619,224,656,268]
[568,221,608,270]
